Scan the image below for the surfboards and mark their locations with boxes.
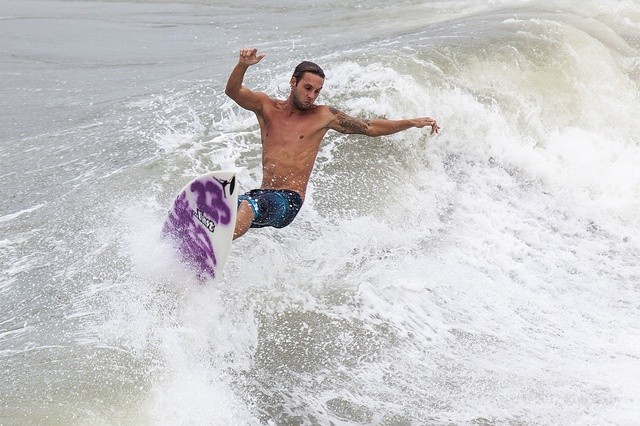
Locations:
[160,169,238,285]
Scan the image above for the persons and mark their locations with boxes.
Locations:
[225,48,440,241]
